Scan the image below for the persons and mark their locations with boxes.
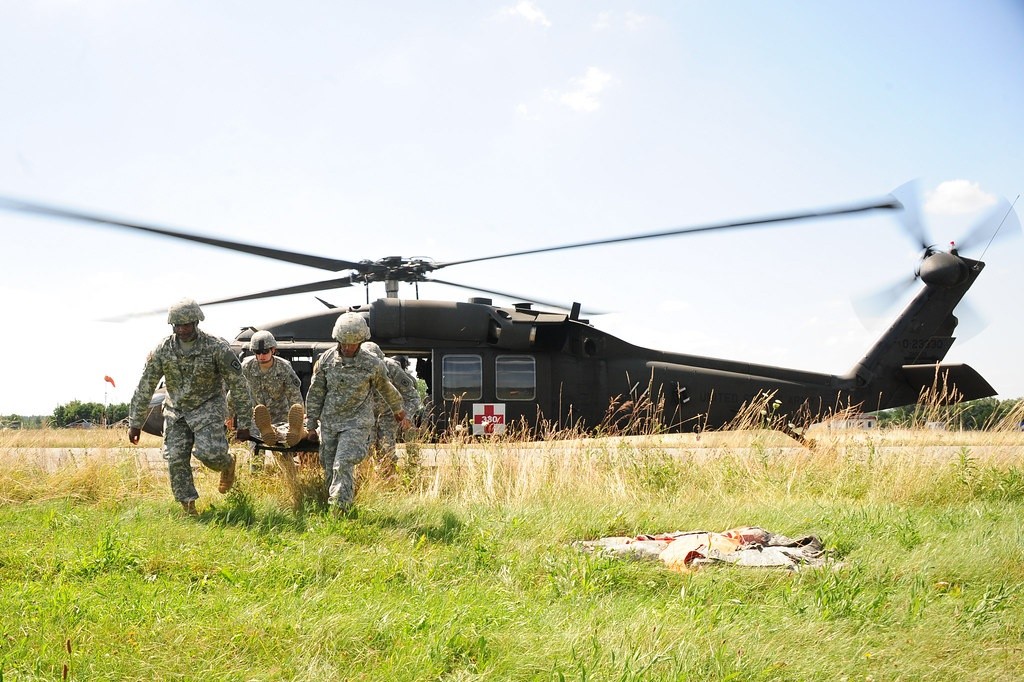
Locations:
[251,403,322,446]
[389,354,418,441]
[127,299,254,518]
[305,312,413,521]
[358,342,424,485]
[225,330,305,476]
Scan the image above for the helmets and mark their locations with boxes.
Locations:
[360,342,385,359]
[332,313,371,344]
[251,331,277,351]
[167,299,205,324]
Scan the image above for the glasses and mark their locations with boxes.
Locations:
[254,349,269,354]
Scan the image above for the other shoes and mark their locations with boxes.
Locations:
[219,454,237,494]
[254,405,277,445]
[286,403,308,447]
[182,501,198,516]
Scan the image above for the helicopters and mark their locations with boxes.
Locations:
[97,198,1000,453]
